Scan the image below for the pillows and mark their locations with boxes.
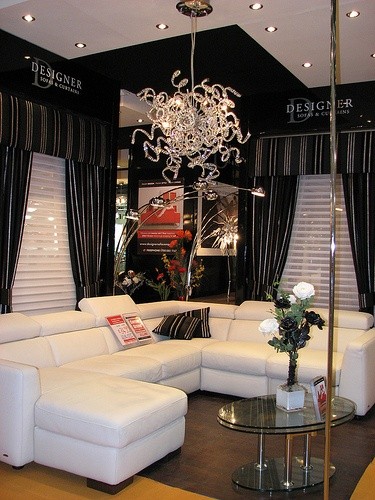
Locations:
[152,316,202,340]
[172,307,212,337]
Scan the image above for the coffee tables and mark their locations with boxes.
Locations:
[217,393,357,493]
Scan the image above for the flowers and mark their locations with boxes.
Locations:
[259,276,325,384]
[164,231,204,294]
[146,273,172,301]
[114,270,147,296]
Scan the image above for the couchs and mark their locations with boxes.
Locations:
[1,294,374,495]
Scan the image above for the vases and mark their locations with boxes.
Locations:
[178,292,186,302]
[278,383,307,413]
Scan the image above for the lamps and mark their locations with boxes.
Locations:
[113,181,266,299]
[131,0,251,186]
